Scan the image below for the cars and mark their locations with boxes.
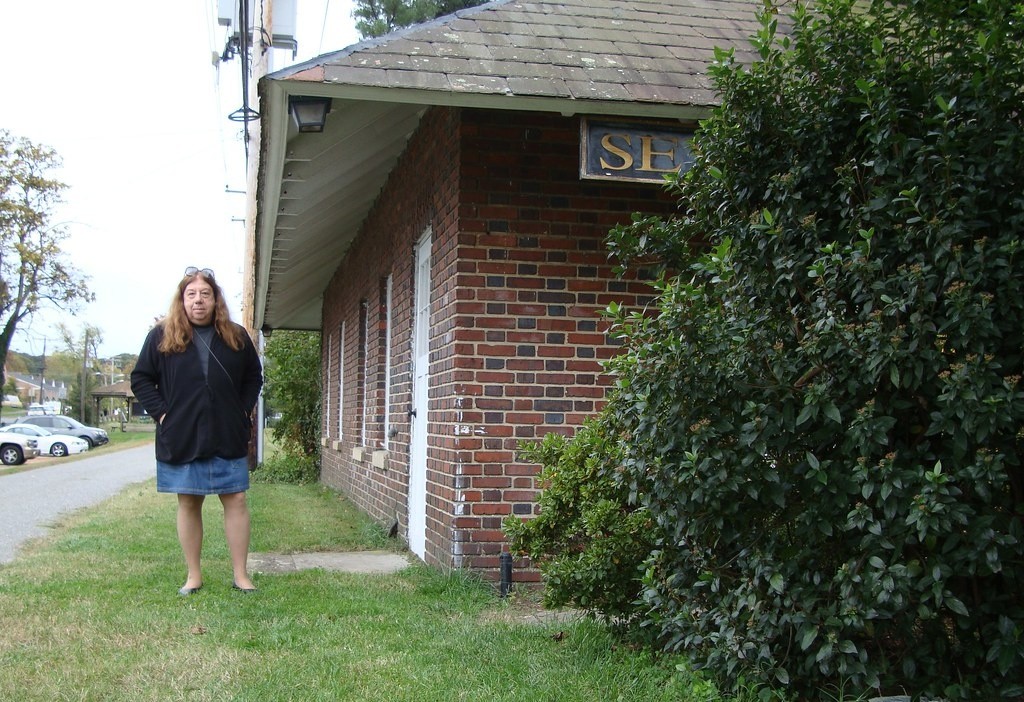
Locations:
[26,402,73,416]
[0,431,42,465]
[0,424,89,458]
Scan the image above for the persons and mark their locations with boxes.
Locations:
[131,267,264,596]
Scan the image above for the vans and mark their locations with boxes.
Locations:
[1,394,23,408]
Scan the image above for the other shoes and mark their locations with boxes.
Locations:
[179,587,200,595]
[232,582,257,592]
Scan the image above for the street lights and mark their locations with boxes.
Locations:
[109,379,125,421]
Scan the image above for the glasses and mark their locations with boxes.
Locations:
[184,267,214,281]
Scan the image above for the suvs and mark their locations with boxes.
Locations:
[13,414,109,451]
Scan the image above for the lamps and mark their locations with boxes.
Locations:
[286,94,332,135]
